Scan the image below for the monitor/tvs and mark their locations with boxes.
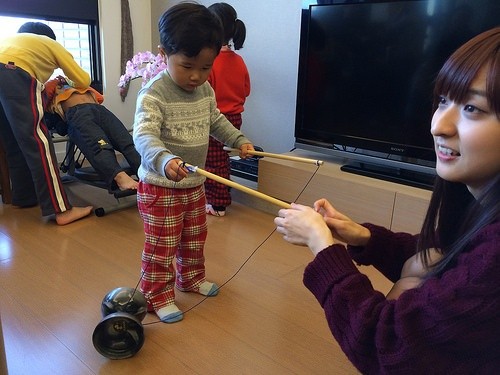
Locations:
[295,0,500,191]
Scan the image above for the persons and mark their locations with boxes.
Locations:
[0,22,93,225]
[133,3,255,324]
[274,25,500,375]
[41,75,141,194]
[205,3,251,217]
